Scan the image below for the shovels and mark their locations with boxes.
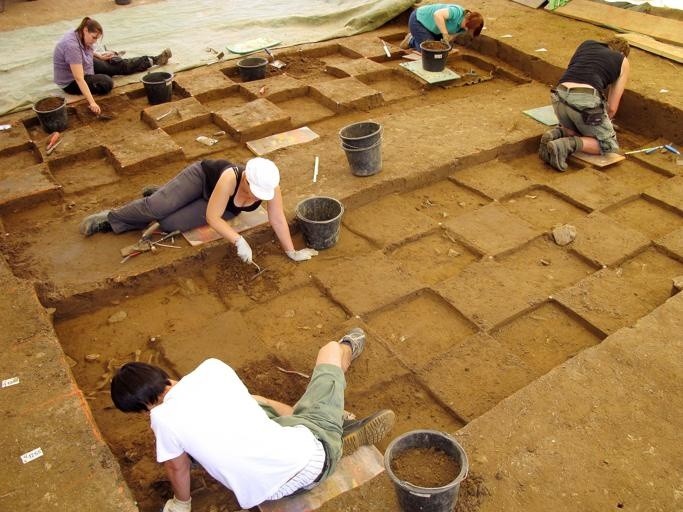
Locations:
[120,222,160,257]
[266,48,287,68]
[195,131,226,147]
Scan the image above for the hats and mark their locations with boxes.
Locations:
[245,157,280,201]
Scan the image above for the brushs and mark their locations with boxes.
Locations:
[45,131,59,150]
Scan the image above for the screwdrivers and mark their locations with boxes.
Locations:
[153,230,180,244]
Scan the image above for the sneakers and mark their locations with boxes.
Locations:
[338,328,366,361]
[342,408,398,459]
[158,47,172,65]
[538,123,574,172]
[81,209,113,237]
[399,33,413,49]
[142,184,160,197]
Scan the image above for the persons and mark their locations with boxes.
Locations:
[80,154,319,265]
[400,3,483,53]
[110,328,396,512]
[536,36,630,171]
[54,16,173,114]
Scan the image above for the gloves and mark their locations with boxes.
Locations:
[285,248,319,262]
[440,32,450,46]
[162,494,192,512]
[234,235,253,265]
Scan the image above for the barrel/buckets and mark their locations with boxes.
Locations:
[383,428,470,512]
[236,56,269,83]
[340,143,383,177]
[338,120,382,150]
[32,95,69,134]
[294,195,345,252]
[420,39,452,72]
[141,71,174,105]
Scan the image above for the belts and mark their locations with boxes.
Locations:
[557,82,600,97]
